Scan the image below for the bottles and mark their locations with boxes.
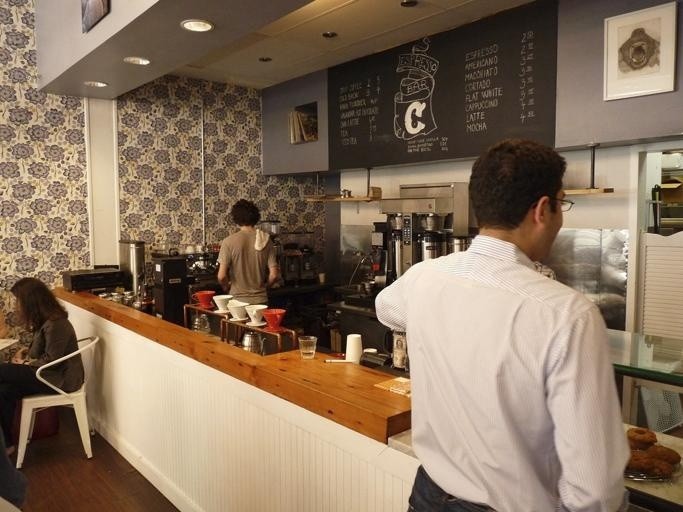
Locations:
[242,331,266,356]
[97,289,141,308]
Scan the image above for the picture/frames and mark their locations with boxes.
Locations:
[603,1,676,101]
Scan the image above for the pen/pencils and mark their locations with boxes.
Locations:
[324,360,356,363]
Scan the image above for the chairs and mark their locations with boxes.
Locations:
[16,336,100,470]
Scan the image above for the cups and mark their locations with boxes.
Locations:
[192,290,287,332]
[297,336,318,360]
[346,333,363,363]
[318,272,325,283]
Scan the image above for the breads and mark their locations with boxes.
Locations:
[625,427,681,477]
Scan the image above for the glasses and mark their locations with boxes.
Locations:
[532,196,573,214]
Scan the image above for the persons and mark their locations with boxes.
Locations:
[0,277,84,456]
[375,140,629,512]
[216,197,281,321]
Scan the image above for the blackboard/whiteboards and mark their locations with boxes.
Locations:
[327,0,559,171]
